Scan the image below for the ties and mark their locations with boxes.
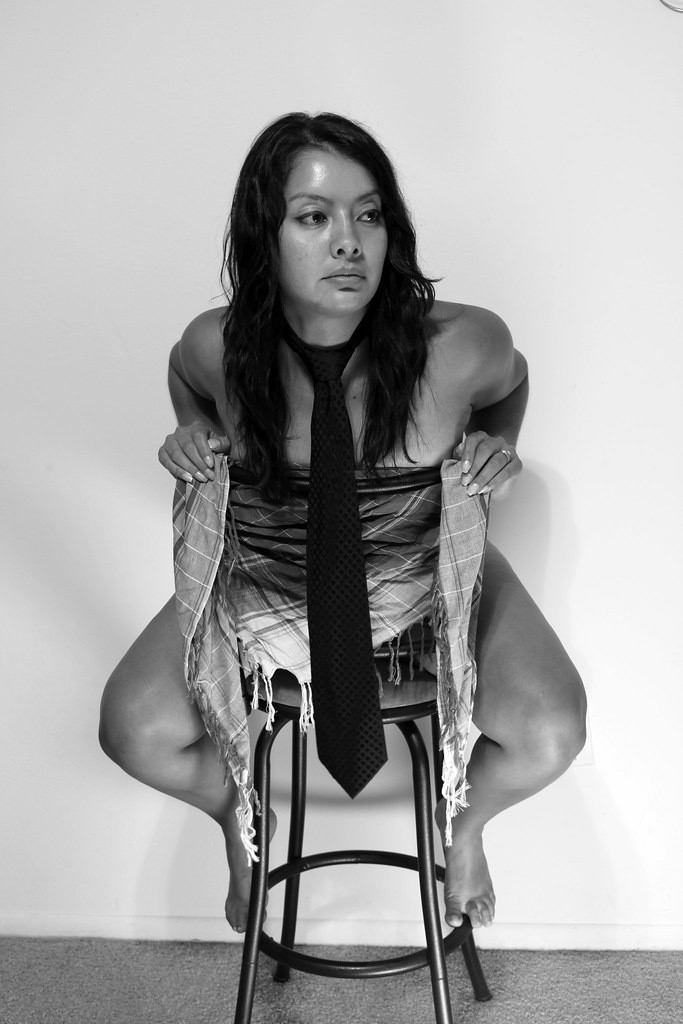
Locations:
[294,343,389,798]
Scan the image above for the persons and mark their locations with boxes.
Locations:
[98,114,587,933]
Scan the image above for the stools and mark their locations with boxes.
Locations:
[233,656,492,1024]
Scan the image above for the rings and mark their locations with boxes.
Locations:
[499,450,511,462]
